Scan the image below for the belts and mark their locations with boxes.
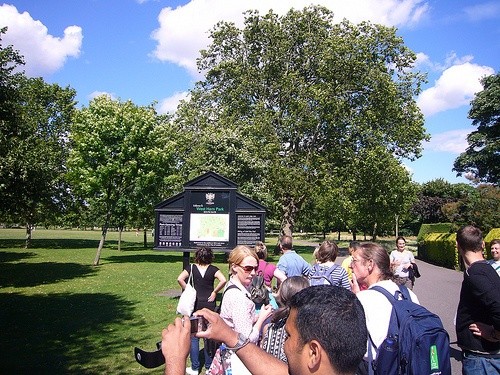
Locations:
[462,350,500,359]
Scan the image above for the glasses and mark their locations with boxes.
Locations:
[491,248,500,250]
[237,264,258,271]
[351,259,363,265]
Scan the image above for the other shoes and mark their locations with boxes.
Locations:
[186,367,198,375]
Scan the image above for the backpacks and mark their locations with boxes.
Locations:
[247,262,269,306]
[309,263,339,287]
[204,285,250,361]
[365,284,452,375]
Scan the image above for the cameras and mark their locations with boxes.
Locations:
[181,316,202,334]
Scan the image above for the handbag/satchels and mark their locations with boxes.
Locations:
[176,264,197,318]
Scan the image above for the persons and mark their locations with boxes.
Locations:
[489,238,500,277]
[161,235,420,375]
[455,225,500,375]
[135,227,155,237]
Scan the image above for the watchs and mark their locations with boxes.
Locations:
[232,333,248,352]
